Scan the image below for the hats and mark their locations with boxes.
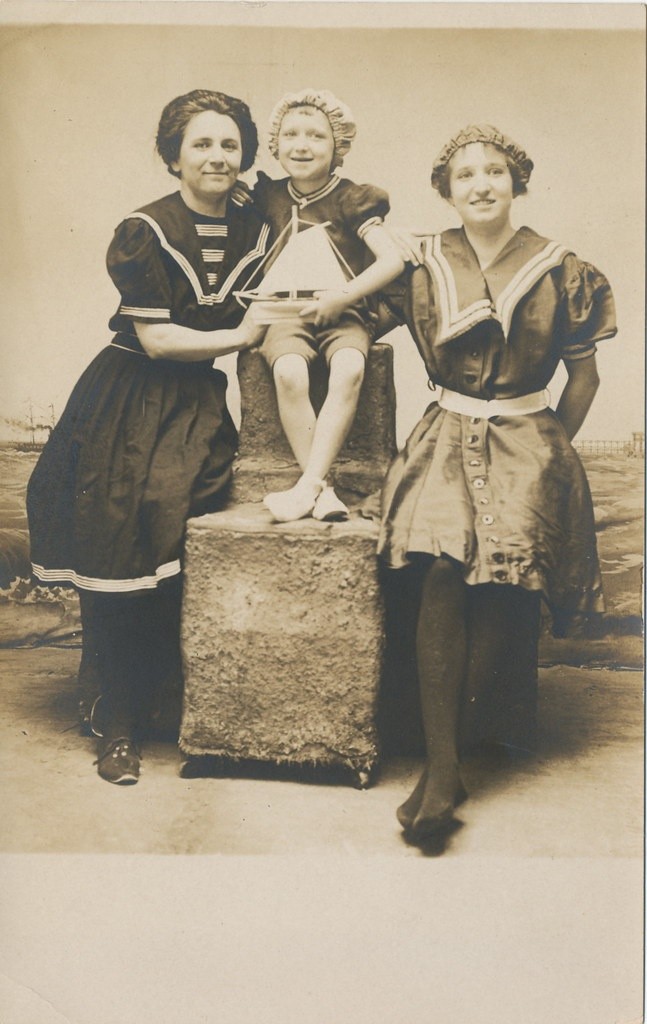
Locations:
[268,89,356,175]
[431,122,534,199]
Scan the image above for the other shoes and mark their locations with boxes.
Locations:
[92,736,142,783]
[312,480,350,521]
[78,691,107,736]
[396,767,466,836]
[263,478,317,521]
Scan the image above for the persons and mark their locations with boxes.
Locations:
[232,92,404,523]
[376,123,619,834]
[28,88,274,786]
